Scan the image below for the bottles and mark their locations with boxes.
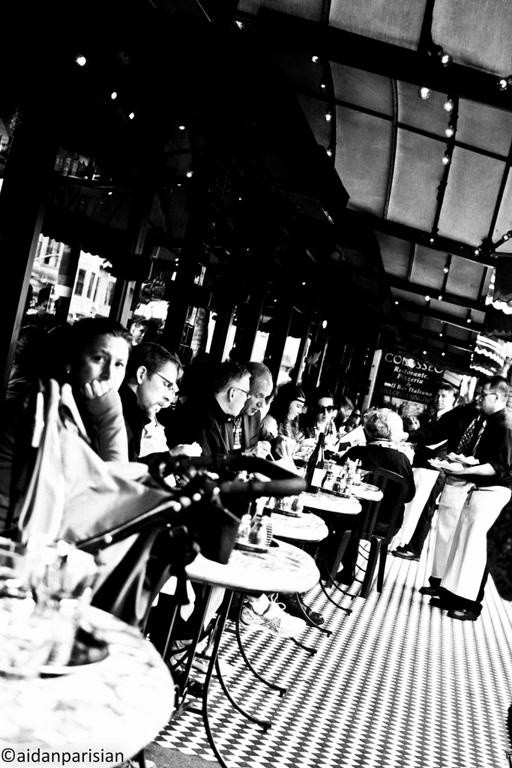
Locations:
[242,507,273,545]
[304,432,326,494]
[323,471,351,498]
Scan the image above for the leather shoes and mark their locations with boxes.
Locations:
[418,585,481,626]
[391,541,424,563]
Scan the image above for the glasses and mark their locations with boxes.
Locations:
[156,373,173,391]
[317,406,334,412]
[236,387,252,400]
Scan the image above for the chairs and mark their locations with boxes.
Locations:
[327,465,406,598]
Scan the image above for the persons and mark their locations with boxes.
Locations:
[13,316,511,646]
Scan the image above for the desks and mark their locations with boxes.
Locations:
[0,465,386,768]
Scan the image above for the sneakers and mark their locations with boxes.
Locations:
[275,595,324,628]
[334,566,357,588]
[239,600,308,639]
